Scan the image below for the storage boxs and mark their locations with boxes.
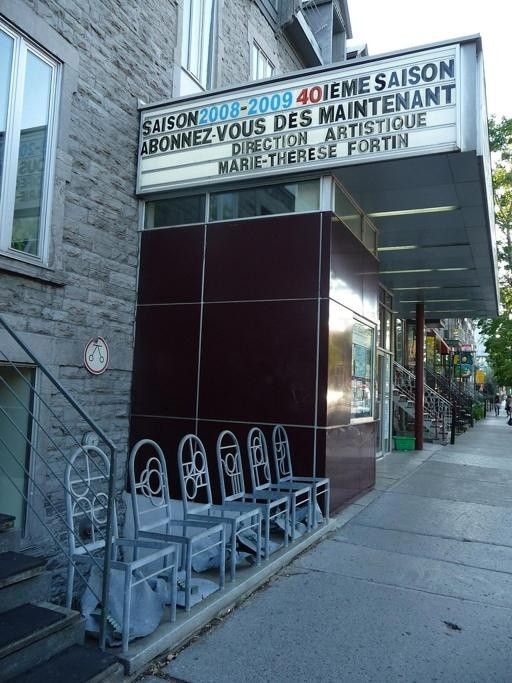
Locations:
[393,435,416,451]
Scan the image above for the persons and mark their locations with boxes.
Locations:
[506,395,512,419]
[494,396,499,415]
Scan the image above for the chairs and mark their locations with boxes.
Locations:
[177,432,265,582]
[246,426,311,546]
[128,438,227,612]
[66,442,181,653]
[271,422,332,529]
[216,429,292,560]
